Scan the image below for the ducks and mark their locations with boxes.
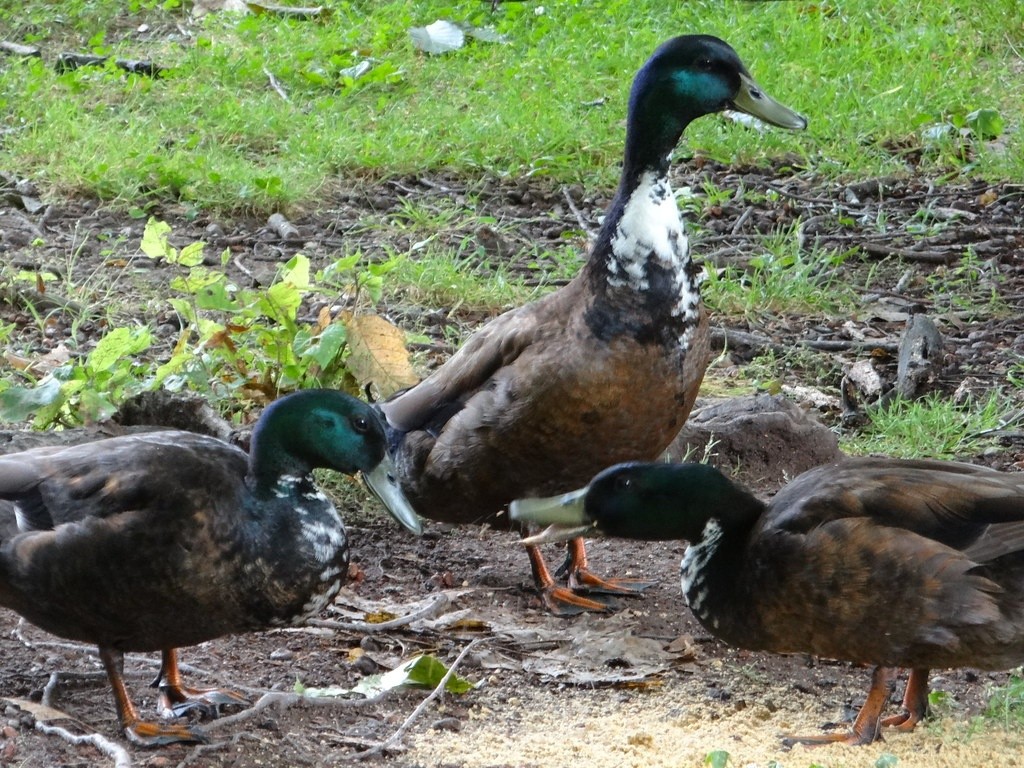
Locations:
[0,390,425,751]
[506,455,1024,750]
[358,34,812,618]
